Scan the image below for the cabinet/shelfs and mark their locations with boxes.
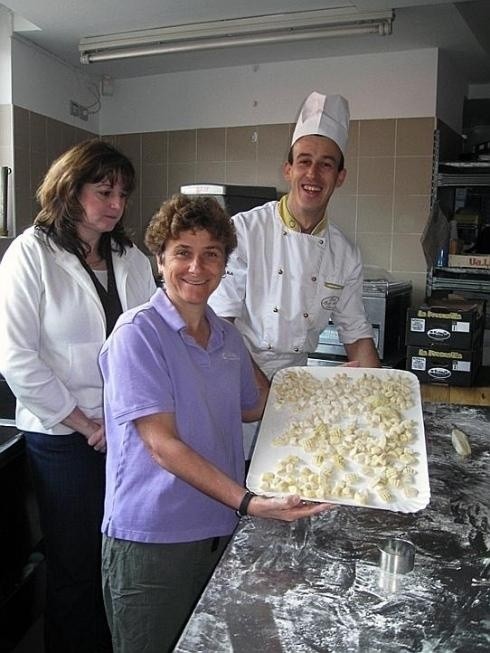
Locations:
[425,128,490,307]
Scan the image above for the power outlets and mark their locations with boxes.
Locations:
[70,100,89,122]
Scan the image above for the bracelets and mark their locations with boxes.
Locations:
[235,490,257,517]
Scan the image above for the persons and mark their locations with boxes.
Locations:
[1,134,164,653]
[95,193,362,653]
[196,134,384,489]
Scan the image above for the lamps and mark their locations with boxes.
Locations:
[78,4,396,65]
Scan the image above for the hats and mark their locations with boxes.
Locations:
[291,90,350,160]
